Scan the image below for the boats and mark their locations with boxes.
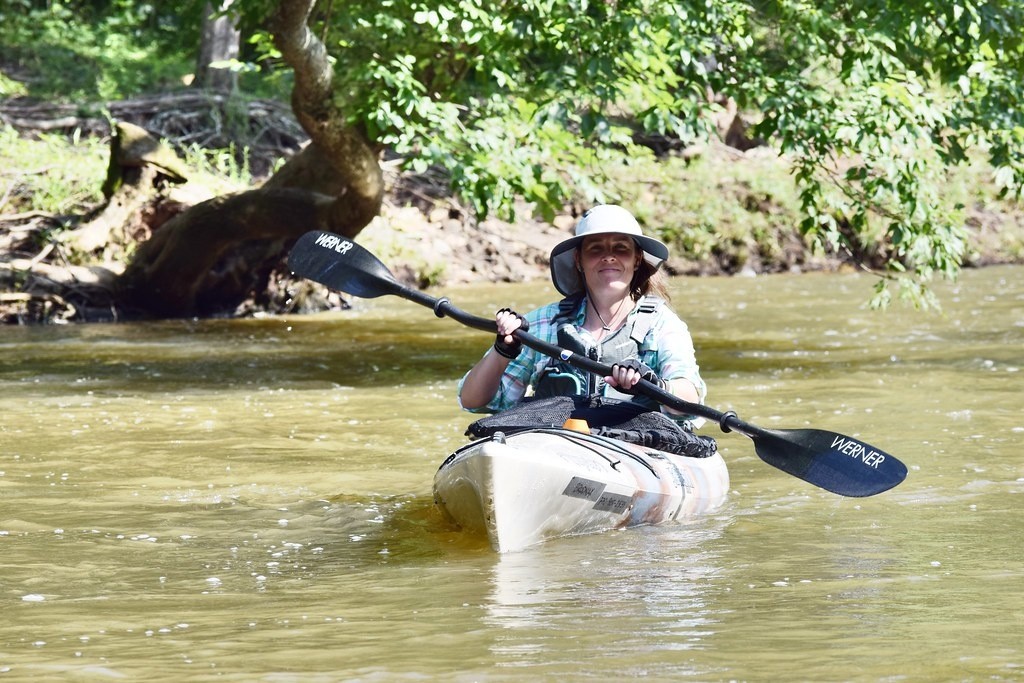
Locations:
[433,422,731,559]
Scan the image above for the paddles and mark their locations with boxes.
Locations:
[286,228,909,499]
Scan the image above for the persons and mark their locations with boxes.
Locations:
[458,205,709,431]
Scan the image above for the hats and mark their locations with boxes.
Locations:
[550,204,668,297]
